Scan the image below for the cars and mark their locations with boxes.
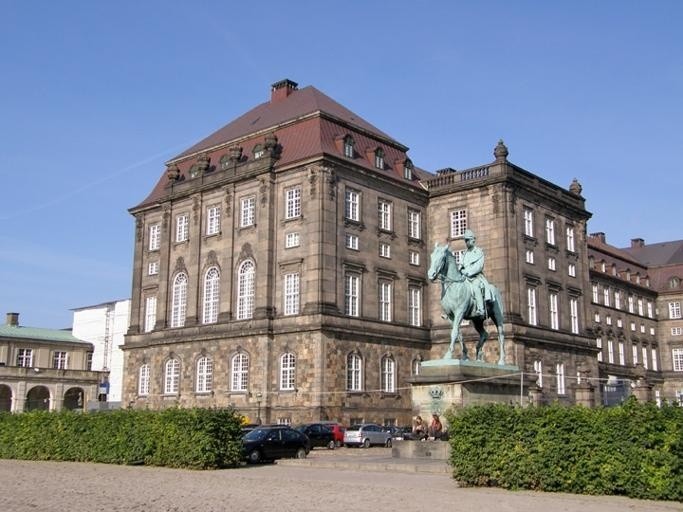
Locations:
[237,422,413,466]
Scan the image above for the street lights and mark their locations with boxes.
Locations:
[253,392,264,424]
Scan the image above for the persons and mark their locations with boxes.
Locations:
[439,229,490,319]
[426,413,442,441]
[413,415,428,441]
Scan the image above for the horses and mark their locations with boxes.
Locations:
[427,240,506,367]
